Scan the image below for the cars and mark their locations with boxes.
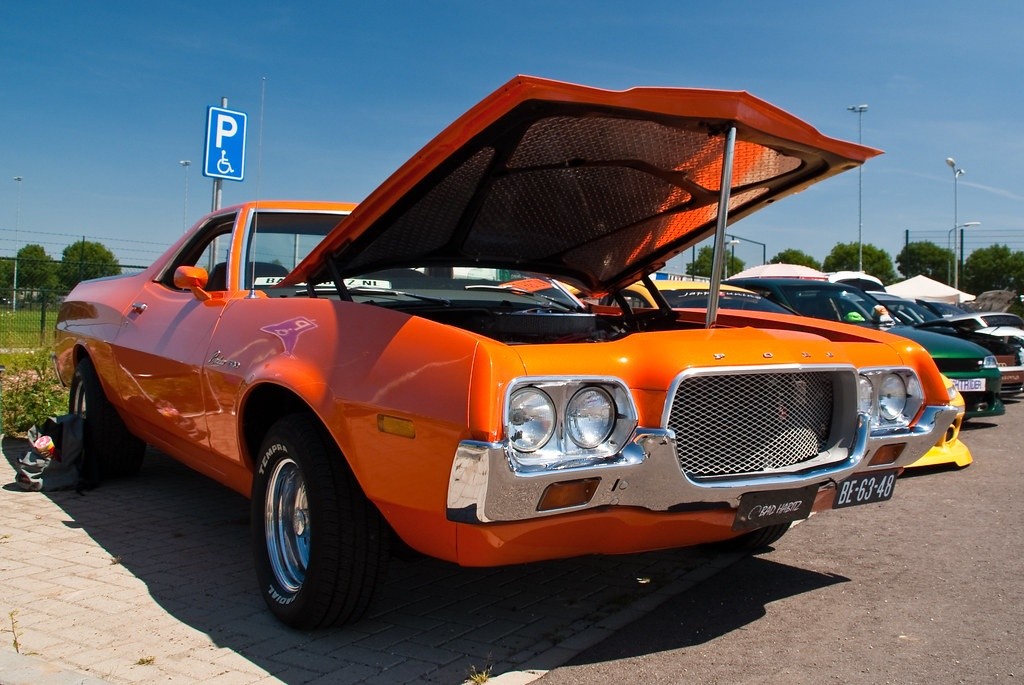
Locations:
[816,270,1023,395]
[726,277,1005,424]
[53,72,960,635]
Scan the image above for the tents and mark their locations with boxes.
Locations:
[884,275,976,303]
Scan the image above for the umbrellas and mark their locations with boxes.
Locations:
[727,263,830,279]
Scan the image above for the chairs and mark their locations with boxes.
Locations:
[204,262,288,291]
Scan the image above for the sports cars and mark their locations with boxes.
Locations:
[553,280,975,475]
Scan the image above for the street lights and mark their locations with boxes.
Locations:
[847,104,872,271]
[180,160,191,237]
[949,221,976,284]
[946,158,967,292]
[13,176,24,309]
